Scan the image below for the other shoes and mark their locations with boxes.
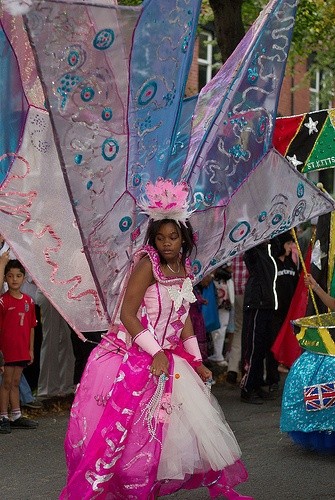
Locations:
[269,382,279,392]
[0,416,12,435]
[239,390,273,404]
[25,399,43,408]
[227,371,237,384]
[10,415,38,429]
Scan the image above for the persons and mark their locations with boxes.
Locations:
[301,269,335,315]
[0,260,38,435]
[0,235,108,411]
[58,212,253,500]
[187,210,335,404]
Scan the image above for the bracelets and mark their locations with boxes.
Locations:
[314,283,319,290]
